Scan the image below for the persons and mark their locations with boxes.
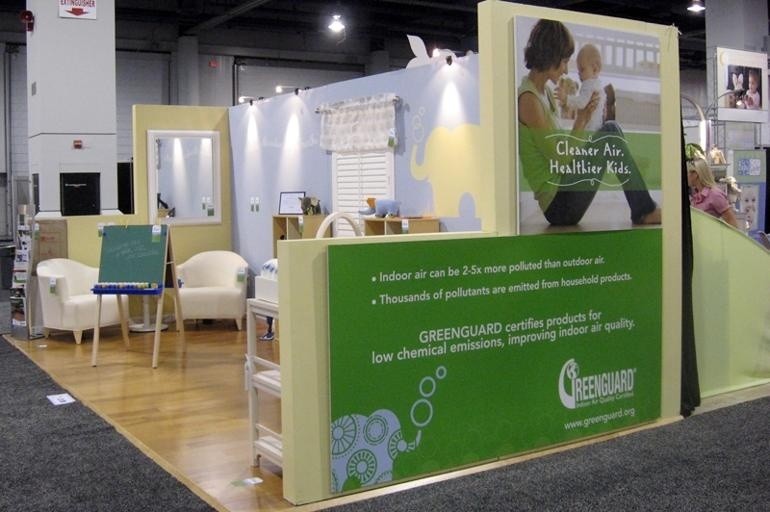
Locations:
[740,185,757,230]
[743,69,760,110]
[686,150,739,229]
[518,18,661,226]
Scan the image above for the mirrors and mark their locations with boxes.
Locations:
[146,129,221,227]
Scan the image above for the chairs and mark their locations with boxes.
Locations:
[37,258,130,344]
[174,250,248,330]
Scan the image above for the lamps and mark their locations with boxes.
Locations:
[238,95,255,104]
[326,0,345,33]
[276,84,299,95]
[432,47,465,60]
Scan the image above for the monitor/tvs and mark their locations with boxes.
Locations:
[116,162,134,213]
[60,172,100,216]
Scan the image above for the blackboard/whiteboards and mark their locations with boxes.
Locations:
[91,224,169,294]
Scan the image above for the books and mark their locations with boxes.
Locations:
[10,204,36,326]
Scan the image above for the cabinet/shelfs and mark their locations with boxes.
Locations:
[245,299,283,477]
[272,215,331,258]
[362,216,442,236]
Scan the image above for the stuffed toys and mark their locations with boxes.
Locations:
[357,197,402,218]
[297,196,321,215]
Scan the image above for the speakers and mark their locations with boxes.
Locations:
[0,1,26,47]
